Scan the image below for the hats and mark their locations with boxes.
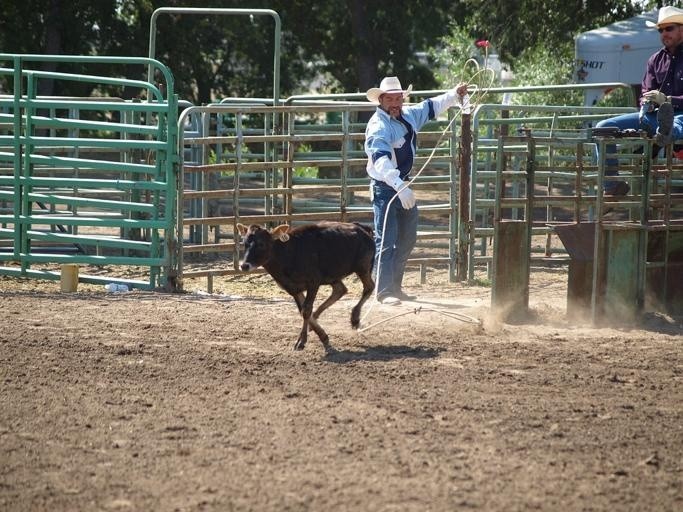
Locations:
[646,6,683,28]
[366,77,413,104]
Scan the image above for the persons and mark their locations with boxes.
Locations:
[593,5,683,216]
[365,76,469,302]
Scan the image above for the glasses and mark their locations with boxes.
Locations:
[658,25,677,33]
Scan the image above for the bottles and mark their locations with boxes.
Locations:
[503,92,511,107]
[462,92,470,115]
[104,283,128,292]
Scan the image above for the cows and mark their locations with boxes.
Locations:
[235,220,378,352]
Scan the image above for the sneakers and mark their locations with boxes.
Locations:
[655,103,675,148]
[395,292,417,300]
[378,296,400,306]
[603,182,630,214]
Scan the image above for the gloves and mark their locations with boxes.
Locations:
[643,90,670,105]
[397,185,416,210]
